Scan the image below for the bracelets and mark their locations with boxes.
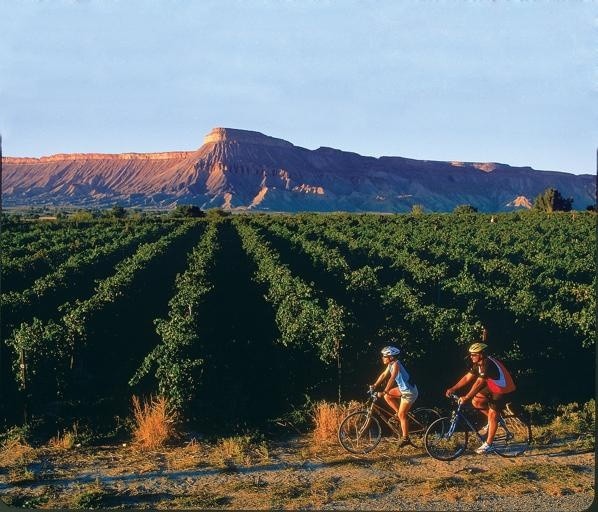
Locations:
[466,395,470,399]
[382,390,387,395]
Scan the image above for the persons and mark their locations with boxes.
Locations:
[445,343,516,454]
[373,346,419,448]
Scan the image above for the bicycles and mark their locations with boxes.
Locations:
[337,385,443,456]
[424,390,533,463]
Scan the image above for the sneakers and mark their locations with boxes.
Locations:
[398,437,410,448]
[478,423,488,435]
[389,414,400,423]
[474,442,495,454]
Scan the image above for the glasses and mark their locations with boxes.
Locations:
[384,356,390,358]
[471,354,479,356]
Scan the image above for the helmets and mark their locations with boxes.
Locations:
[469,343,488,353]
[381,346,401,356]
